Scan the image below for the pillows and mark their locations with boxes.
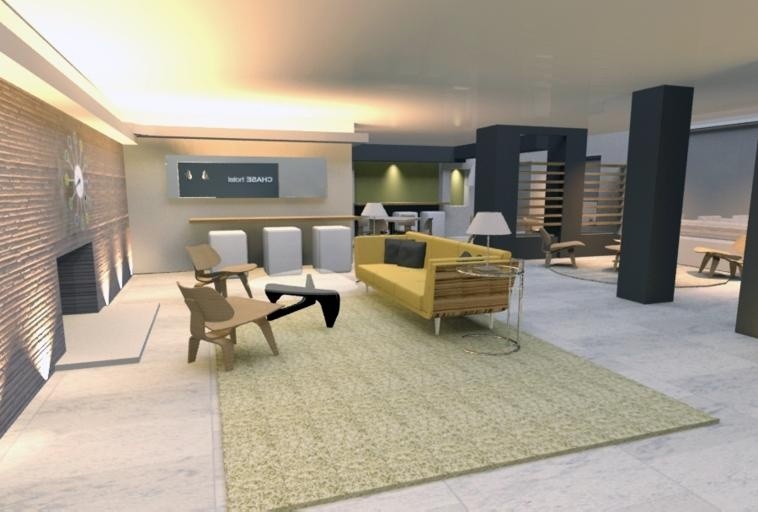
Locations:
[384,239,427,268]
[456,251,483,262]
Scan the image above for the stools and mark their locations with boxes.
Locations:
[312,225,352,273]
[209,230,248,277]
[393,211,445,237]
[262,226,302,276]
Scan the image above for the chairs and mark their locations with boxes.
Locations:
[693,233,746,278]
[185,244,258,298]
[538,227,586,268]
[176,280,285,371]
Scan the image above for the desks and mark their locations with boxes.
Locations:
[606,245,621,272]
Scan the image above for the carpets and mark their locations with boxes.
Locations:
[551,256,729,287]
[216,291,720,512]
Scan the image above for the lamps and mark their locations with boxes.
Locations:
[361,202,389,235]
[466,211,512,271]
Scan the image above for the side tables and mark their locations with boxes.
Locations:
[456,264,524,355]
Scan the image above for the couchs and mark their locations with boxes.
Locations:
[355,231,520,335]
